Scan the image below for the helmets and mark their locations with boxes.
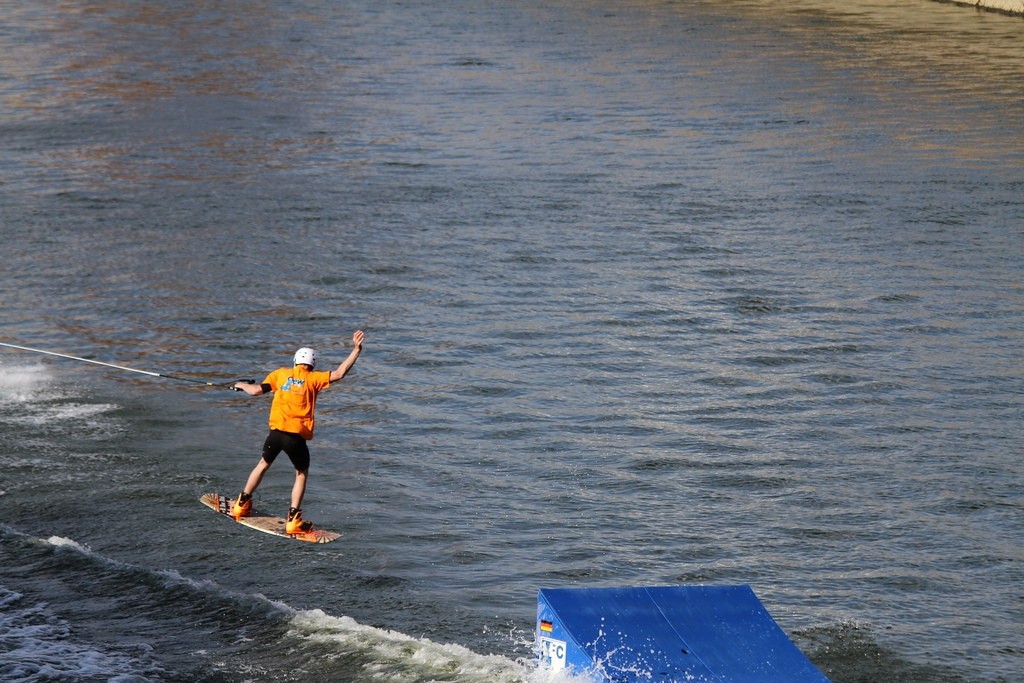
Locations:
[293,347,317,367]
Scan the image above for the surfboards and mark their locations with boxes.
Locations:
[201,493,344,546]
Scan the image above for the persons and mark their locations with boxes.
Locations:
[233,330,365,535]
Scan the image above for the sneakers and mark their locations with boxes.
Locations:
[233,493,253,517]
[286,510,314,534]
